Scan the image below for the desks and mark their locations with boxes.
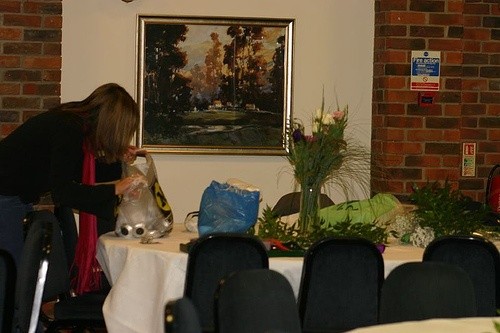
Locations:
[96,223,500,332]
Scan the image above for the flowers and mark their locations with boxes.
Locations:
[279,87,390,222]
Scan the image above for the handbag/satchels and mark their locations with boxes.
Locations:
[198,180,260,237]
[116,149,172,239]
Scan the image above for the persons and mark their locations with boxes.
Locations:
[0,83,140,333]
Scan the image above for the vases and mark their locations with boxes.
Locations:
[298,187,321,234]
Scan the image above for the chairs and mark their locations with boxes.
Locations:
[165,231,500,332]
[1,212,108,333]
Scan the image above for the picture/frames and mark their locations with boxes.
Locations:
[133,14,296,156]
